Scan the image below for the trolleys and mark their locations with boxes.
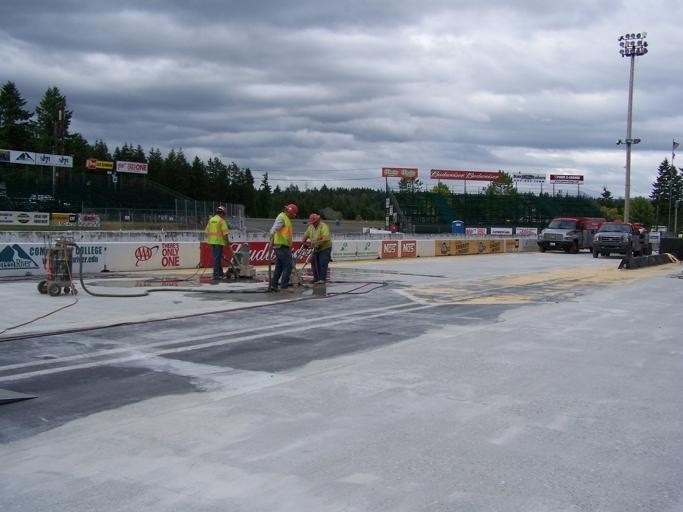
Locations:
[222,242,257,280]
[36,232,78,298]
[275,240,316,293]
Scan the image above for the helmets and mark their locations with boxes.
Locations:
[217,205,227,214]
[284,203,298,218]
[309,213,321,224]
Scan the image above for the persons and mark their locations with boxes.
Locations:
[204,205,230,280]
[266,204,298,291]
[301,211,332,284]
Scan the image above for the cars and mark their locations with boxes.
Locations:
[27,194,71,213]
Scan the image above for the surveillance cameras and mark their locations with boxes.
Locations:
[615,139,622,146]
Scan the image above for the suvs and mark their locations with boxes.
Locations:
[537,216,665,260]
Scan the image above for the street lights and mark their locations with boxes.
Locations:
[615,30,649,226]
